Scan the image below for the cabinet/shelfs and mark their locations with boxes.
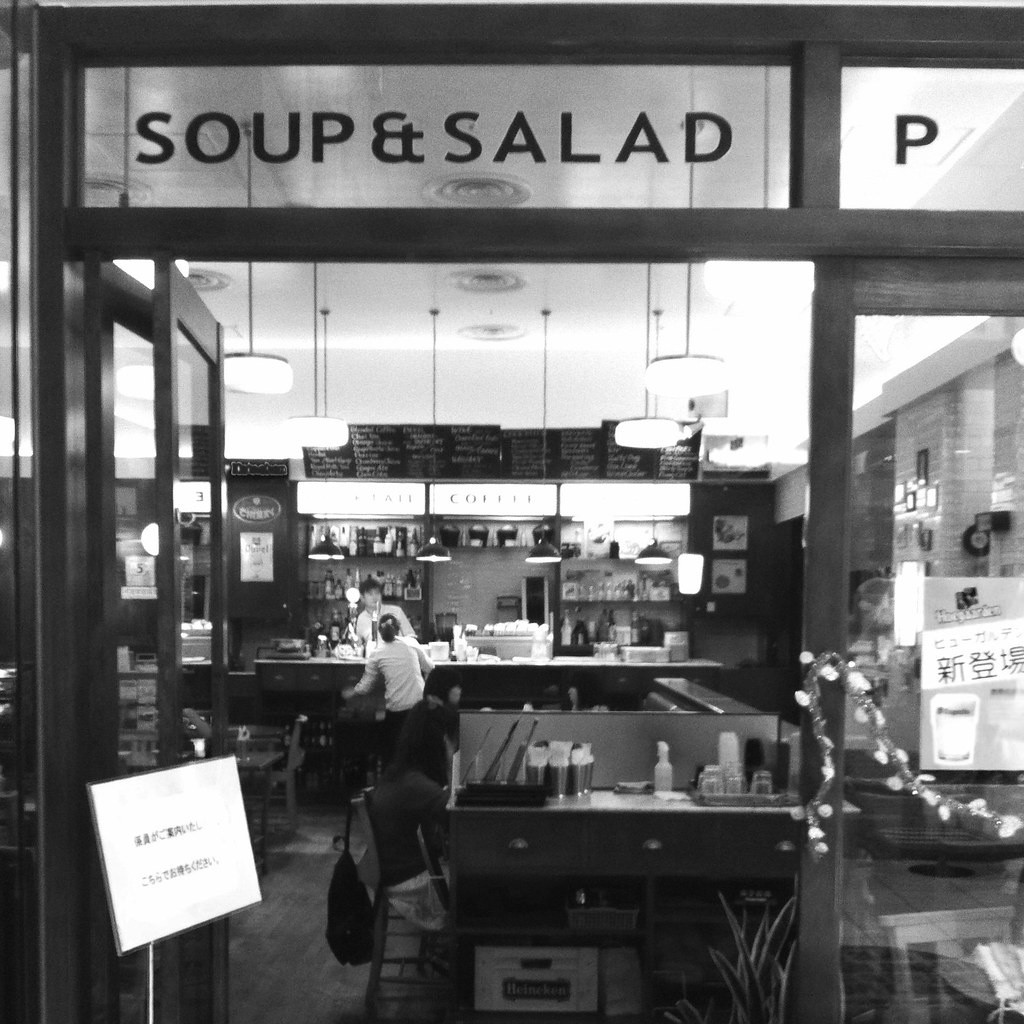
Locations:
[303,512,696,656]
[440,776,806,1023]
[253,660,723,788]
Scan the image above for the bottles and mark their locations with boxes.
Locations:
[309,565,421,599]
[561,610,572,646]
[578,579,636,601]
[311,524,421,558]
[311,606,357,645]
[570,621,589,646]
[609,540,619,560]
[598,609,663,644]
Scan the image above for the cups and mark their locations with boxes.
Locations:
[571,762,593,797]
[550,764,569,797]
[697,732,774,794]
[527,763,547,784]
[435,612,456,642]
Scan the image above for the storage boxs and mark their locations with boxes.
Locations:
[473,945,641,1016]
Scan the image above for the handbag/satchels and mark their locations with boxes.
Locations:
[326,851,372,965]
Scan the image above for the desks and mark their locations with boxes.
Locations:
[228,751,285,874]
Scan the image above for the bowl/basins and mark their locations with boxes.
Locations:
[533,529,554,545]
[497,529,518,548]
[469,530,489,547]
[440,528,460,547]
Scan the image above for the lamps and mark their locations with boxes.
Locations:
[634,308,672,565]
[614,265,681,447]
[113,68,190,290]
[284,263,349,449]
[309,331,333,560]
[529,310,562,563]
[217,121,294,390]
[416,307,451,561]
[642,65,731,394]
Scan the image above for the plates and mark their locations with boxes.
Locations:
[455,778,546,807]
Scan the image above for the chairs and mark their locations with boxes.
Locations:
[360,787,554,1024]
[227,723,308,828]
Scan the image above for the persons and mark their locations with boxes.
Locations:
[342,613,434,738]
[365,696,458,885]
[355,579,417,645]
